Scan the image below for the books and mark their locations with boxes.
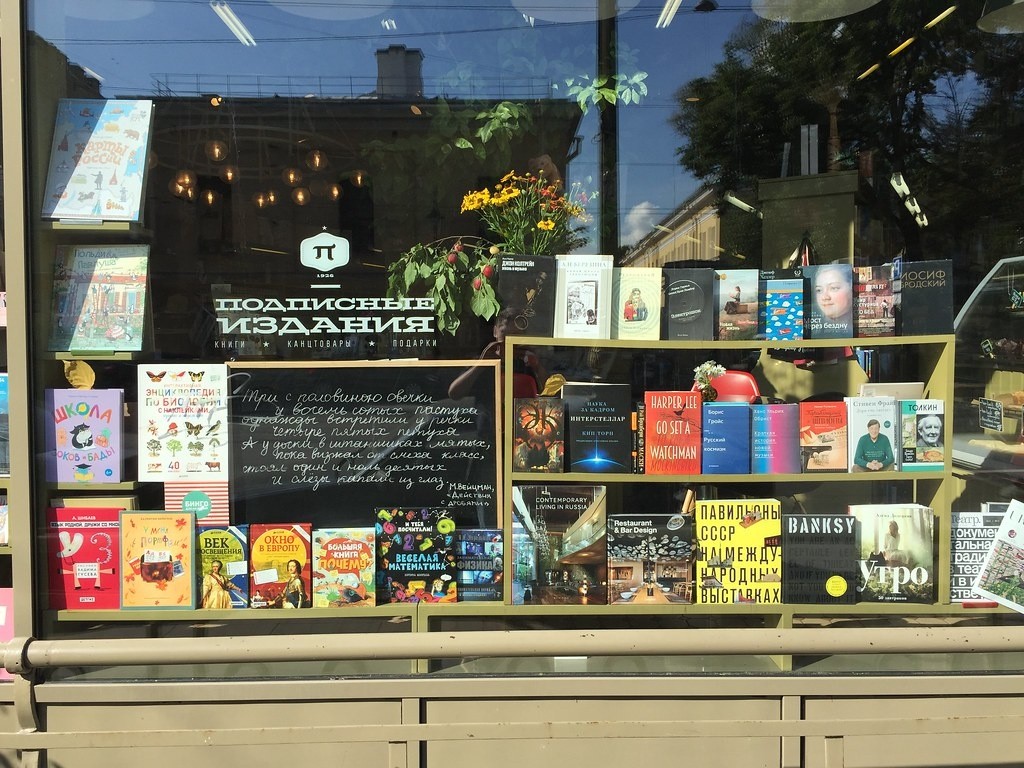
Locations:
[494,252,953,606]
[44,363,506,614]
[0,290,16,685]
[48,243,150,351]
[41,99,156,223]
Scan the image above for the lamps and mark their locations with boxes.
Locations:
[209,0,258,47]
[723,189,762,218]
[889,171,927,227]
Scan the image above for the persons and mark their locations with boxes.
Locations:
[814,264,853,333]
[884,520,906,562]
[851,419,895,473]
[724,286,740,314]
[448,306,549,536]
[917,415,944,447]
[881,300,888,318]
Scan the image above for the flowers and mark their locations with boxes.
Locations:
[692,359,726,389]
[384,169,601,340]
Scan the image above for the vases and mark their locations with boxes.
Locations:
[701,385,718,402]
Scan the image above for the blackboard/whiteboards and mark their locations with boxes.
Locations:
[224,359,503,530]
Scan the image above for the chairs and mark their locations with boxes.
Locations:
[673,358,691,390]
[689,370,760,404]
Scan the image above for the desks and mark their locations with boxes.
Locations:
[715,394,787,404]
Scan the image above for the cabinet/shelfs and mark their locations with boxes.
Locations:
[756,169,909,397]
[31,219,956,623]
